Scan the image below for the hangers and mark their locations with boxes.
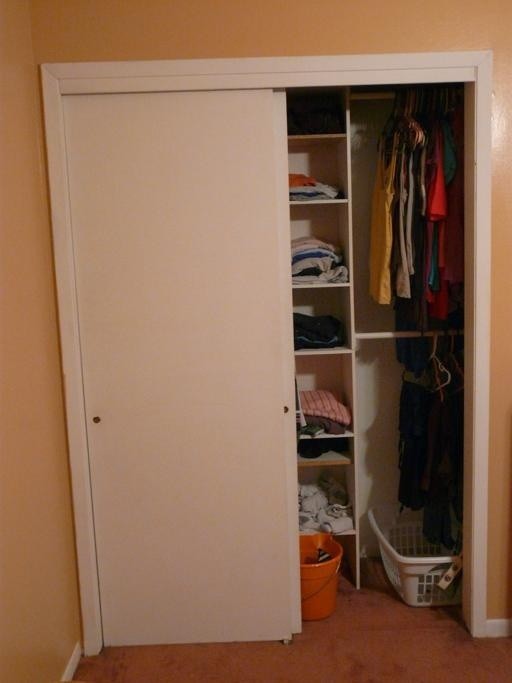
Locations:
[376,87,456,152]
[425,331,463,401]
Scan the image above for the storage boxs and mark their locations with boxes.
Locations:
[369,504,462,607]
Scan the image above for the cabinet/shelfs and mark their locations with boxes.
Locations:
[39,51,494,656]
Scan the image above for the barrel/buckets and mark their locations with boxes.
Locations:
[300,533,343,623]
